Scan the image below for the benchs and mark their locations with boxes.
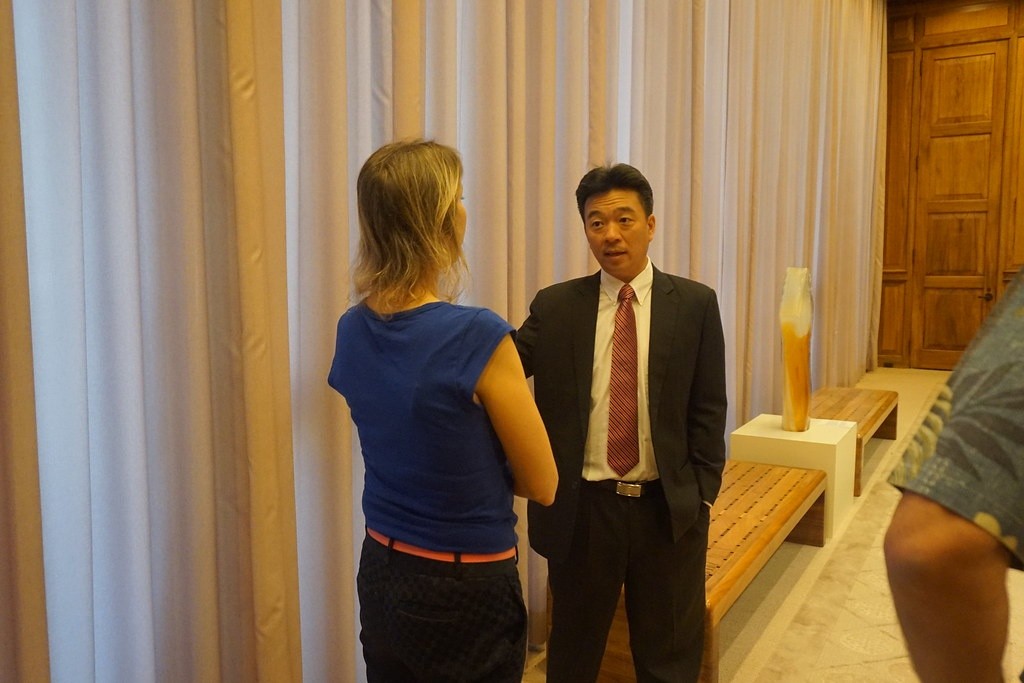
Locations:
[810,386,898,499]
[596,460,828,683]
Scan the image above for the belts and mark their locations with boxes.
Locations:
[581,479,661,498]
[366,528,516,563]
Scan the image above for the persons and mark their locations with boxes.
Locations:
[884,265,1024,683]
[515,163,729,683]
[327,137,558,683]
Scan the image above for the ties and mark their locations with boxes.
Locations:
[606,284,639,477]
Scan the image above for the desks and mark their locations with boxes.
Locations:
[730,413,857,538]
